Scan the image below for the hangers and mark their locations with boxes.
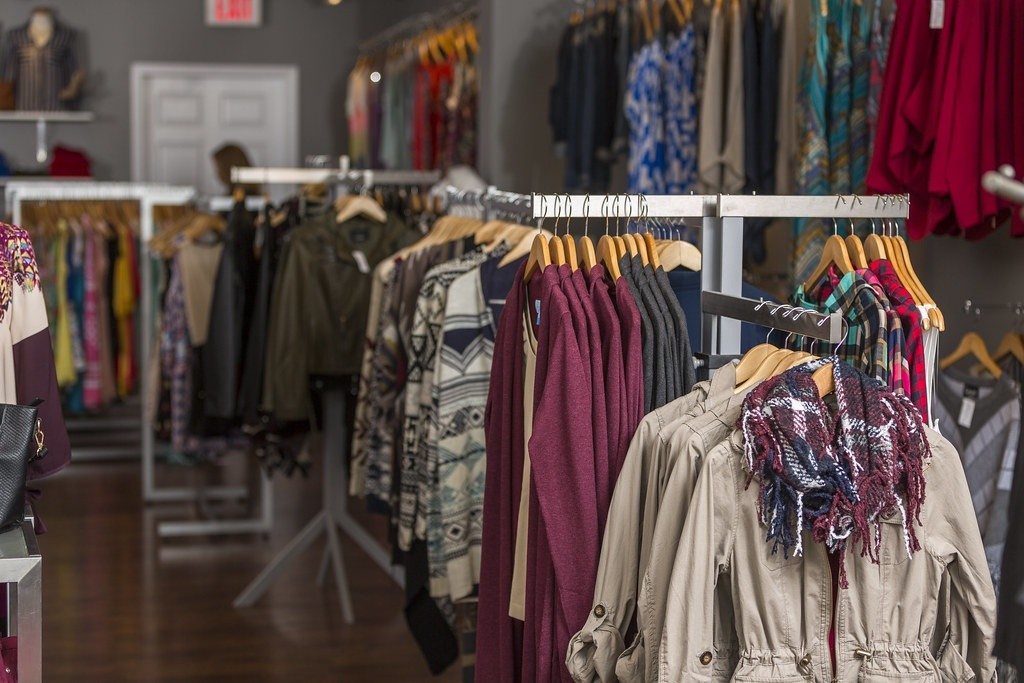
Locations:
[6,157,1024,476]
[329,0,782,104]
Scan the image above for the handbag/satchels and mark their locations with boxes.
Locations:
[0,397,48,534]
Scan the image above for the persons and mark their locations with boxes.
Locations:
[0,8,87,112]
[214,145,263,197]
